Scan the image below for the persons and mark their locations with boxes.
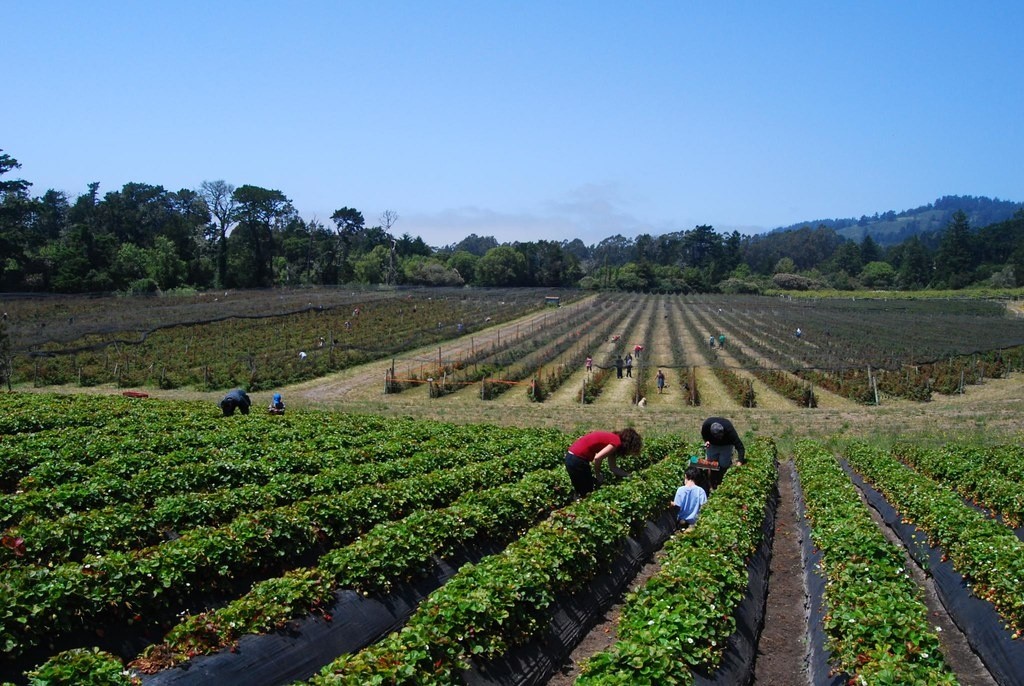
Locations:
[623,352,632,367]
[344,319,353,330]
[634,344,643,358]
[667,466,708,533]
[586,354,593,372]
[787,294,792,301]
[267,394,286,416]
[796,328,802,338]
[709,335,715,349]
[564,427,644,501]
[653,369,665,394]
[297,350,308,361]
[625,359,632,378]
[717,333,725,350]
[456,322,464,333]
[436,321,442,329]
[778,293,785,299]
[217,389,252,417]
[616,355,624,379]
[611,335,620,343]
[700,417,745,490]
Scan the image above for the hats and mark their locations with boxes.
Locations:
[273,393,281,404]
